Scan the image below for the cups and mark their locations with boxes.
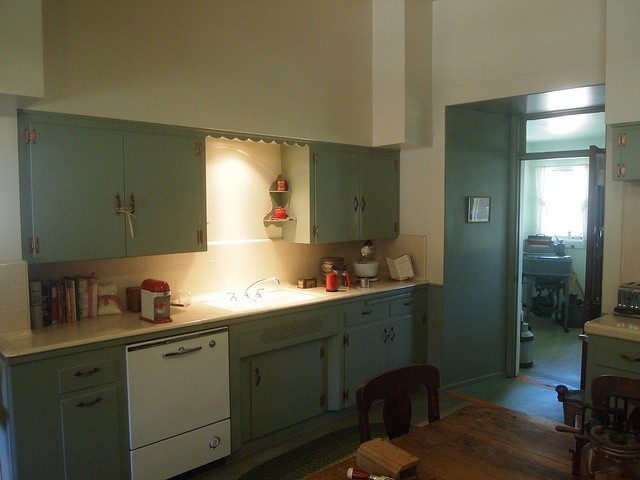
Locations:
[325,272,338,292]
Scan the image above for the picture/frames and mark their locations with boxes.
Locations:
[467,196,491,222]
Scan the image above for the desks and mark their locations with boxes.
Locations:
[299,399,639,476]
[522,253,572,334]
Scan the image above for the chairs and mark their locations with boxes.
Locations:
[358,364,438,438]
[588,374,640,434]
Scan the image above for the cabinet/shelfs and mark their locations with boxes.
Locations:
[229,303,339,462]
[340,283,429,413]
[612,123,639,182]
[2,341,131,476]
[282,137,403,243]
[18,108,208,267]
[585,312,639,436]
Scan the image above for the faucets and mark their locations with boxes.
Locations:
[244,276,280,298]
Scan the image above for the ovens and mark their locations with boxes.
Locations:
[121,327,231,480]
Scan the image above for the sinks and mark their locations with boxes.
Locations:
[203,289,322,313]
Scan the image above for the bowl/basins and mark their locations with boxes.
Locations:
[353,261,380,278]
